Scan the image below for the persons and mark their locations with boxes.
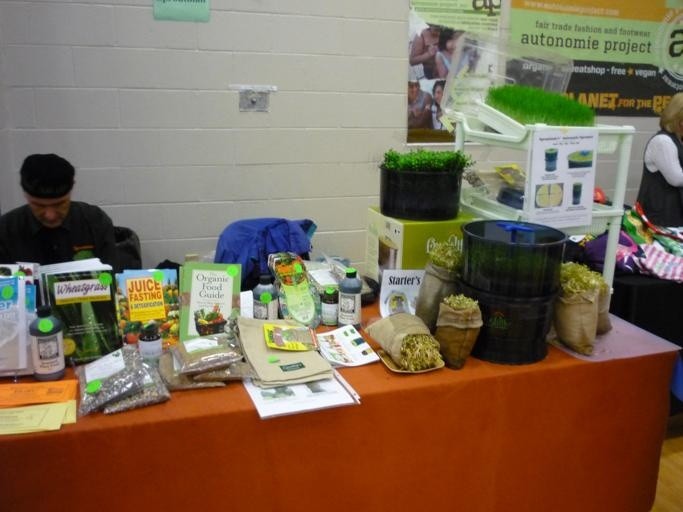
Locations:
[407,22,477,129]
[637,92,683,227]
[0,154,125,275]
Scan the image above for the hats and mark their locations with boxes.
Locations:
[20,152,75,200]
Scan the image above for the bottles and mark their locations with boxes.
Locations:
[251,274,279,321]
[543,147,558,173]
[571,181,584,208]
[137,325,162,360]
[28,304,65,382]
[320,287,339,326]
[339,267,363,328]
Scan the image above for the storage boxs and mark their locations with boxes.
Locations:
[362,204,487,295]
[440,28,574,125]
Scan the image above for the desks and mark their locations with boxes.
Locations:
[0,297,683,512]
[593,269,683,324]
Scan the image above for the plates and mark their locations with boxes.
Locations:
[377,348,446,373]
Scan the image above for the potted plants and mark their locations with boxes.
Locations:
[378,147,477,221]
[476,82,596,138]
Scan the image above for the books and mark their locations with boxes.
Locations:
[0,261,333,388]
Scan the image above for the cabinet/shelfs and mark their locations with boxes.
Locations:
[443,97,636,313]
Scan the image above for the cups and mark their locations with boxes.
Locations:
[377,239,396,284]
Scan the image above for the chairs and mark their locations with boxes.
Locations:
[213,216,318,292]
[113,226,143,273]
